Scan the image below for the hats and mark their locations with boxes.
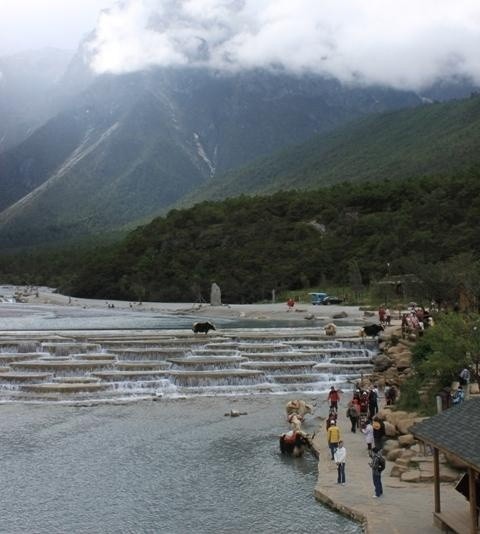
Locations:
[331,419,335,424]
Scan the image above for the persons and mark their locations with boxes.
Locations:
[285,297,295,312]
[325,301,472,499]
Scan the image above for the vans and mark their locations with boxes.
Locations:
[309,292,327,305]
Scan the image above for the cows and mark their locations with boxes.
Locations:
[279,429,309,458]
[358,323,386,341]
[285,399,317,421]
[323,323,336,336]
[190,320,217,335]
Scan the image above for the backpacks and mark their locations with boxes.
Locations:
[375,454,385,472]
[452,391,461,404]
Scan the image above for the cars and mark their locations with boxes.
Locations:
[322,296,343,305]
